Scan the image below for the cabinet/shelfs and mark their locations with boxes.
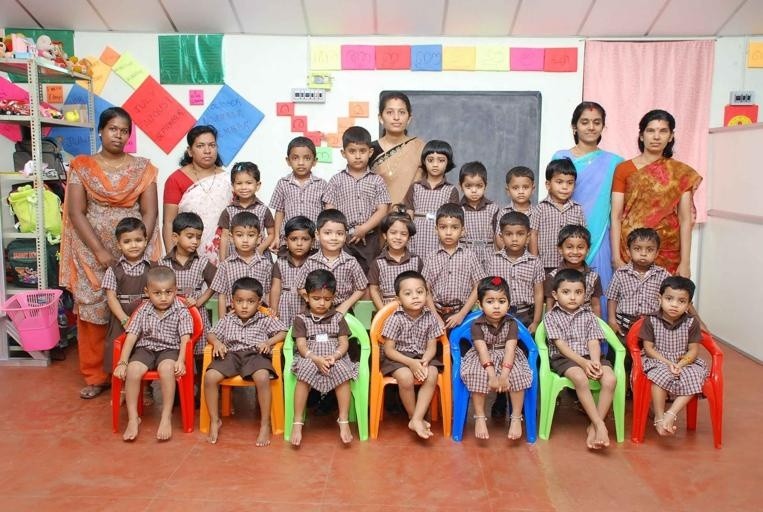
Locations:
[0,56,95,365]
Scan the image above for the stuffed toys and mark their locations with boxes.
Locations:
[0,32,95,79]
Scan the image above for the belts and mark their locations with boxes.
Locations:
[305,333,339,341]
[413,212,436,220]
[485,343,505,351]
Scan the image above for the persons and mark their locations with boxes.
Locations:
[541,266,618,451]
[376,269,447,440]
[98,216,156,407]
[54,104,160,400]
[365,91,427,209]
[201,276,290,448]
[635,275,709,440]
[160,123,231,294]
[550,100,627,362]
[159,210,218,412]
[209,122,543,415]
[541,222,603,417]
[111,263,194,443]
[458,274,532,442]
[609,107,705,281]
[603,226,675,406]
[525,156,587,276]
[286,269,354,447]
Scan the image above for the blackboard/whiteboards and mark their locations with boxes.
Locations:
[379,91,542,223]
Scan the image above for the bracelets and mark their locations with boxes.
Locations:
[681,354,693,364]
[119,316,128,326]
[114,360,127,367]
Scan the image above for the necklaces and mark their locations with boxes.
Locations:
[190,164,217,195]
[574,144,599,165]
[94,151,129,171]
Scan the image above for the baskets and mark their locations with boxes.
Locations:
[0,289,63,352]
